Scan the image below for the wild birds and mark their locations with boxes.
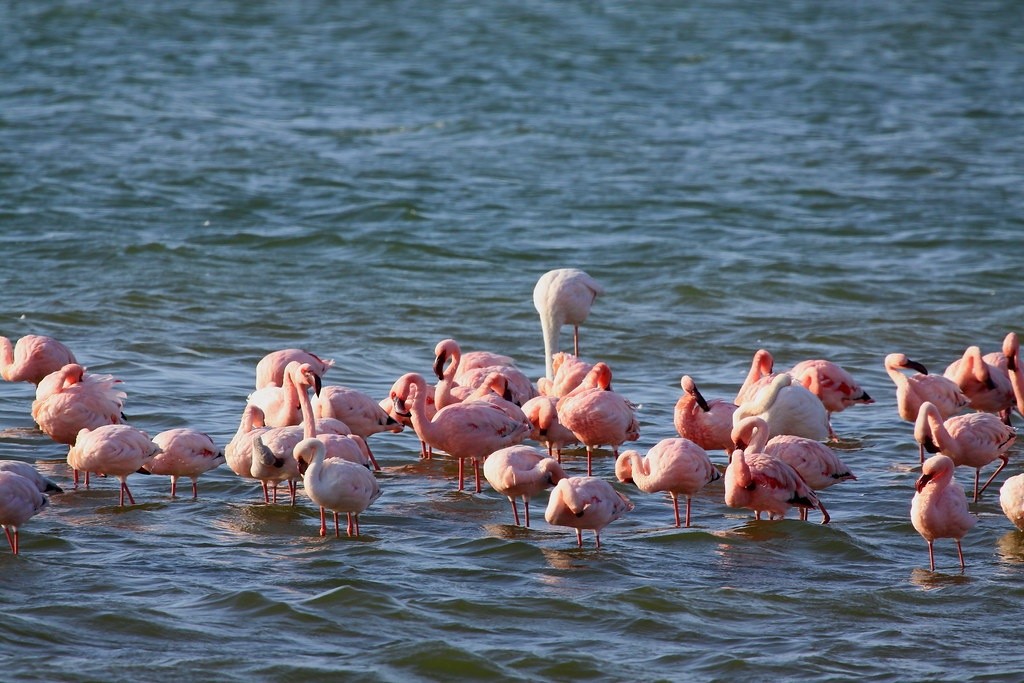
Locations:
[0,269,1024,575]
